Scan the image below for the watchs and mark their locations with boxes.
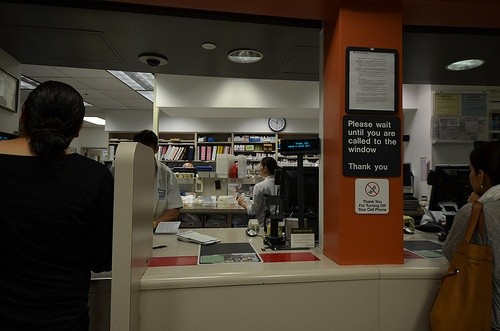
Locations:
[154,221,156,229]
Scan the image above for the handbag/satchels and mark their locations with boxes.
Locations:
[427,201,495,331]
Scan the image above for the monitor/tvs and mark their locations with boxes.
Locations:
[280,166,319,219]
[427,164,473,230]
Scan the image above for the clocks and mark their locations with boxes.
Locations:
[268,118,286,132]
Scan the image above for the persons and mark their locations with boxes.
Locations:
[237,158,286,226]
[0,82,115,331]
[182,163,194,168]
[442,142,500,331]
[133,130,183,232]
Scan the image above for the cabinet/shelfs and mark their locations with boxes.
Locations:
[106,131,278,179]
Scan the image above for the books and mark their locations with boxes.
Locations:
[160,146,194,160]
[198,146,230,161]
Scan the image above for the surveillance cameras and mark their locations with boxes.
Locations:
[138,55,168,68]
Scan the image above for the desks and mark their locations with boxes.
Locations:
[179,200,247,228]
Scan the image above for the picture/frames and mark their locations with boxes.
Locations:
[0,68,19,113]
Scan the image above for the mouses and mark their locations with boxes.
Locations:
[403,226,414,234]
[246,228,257,236]
